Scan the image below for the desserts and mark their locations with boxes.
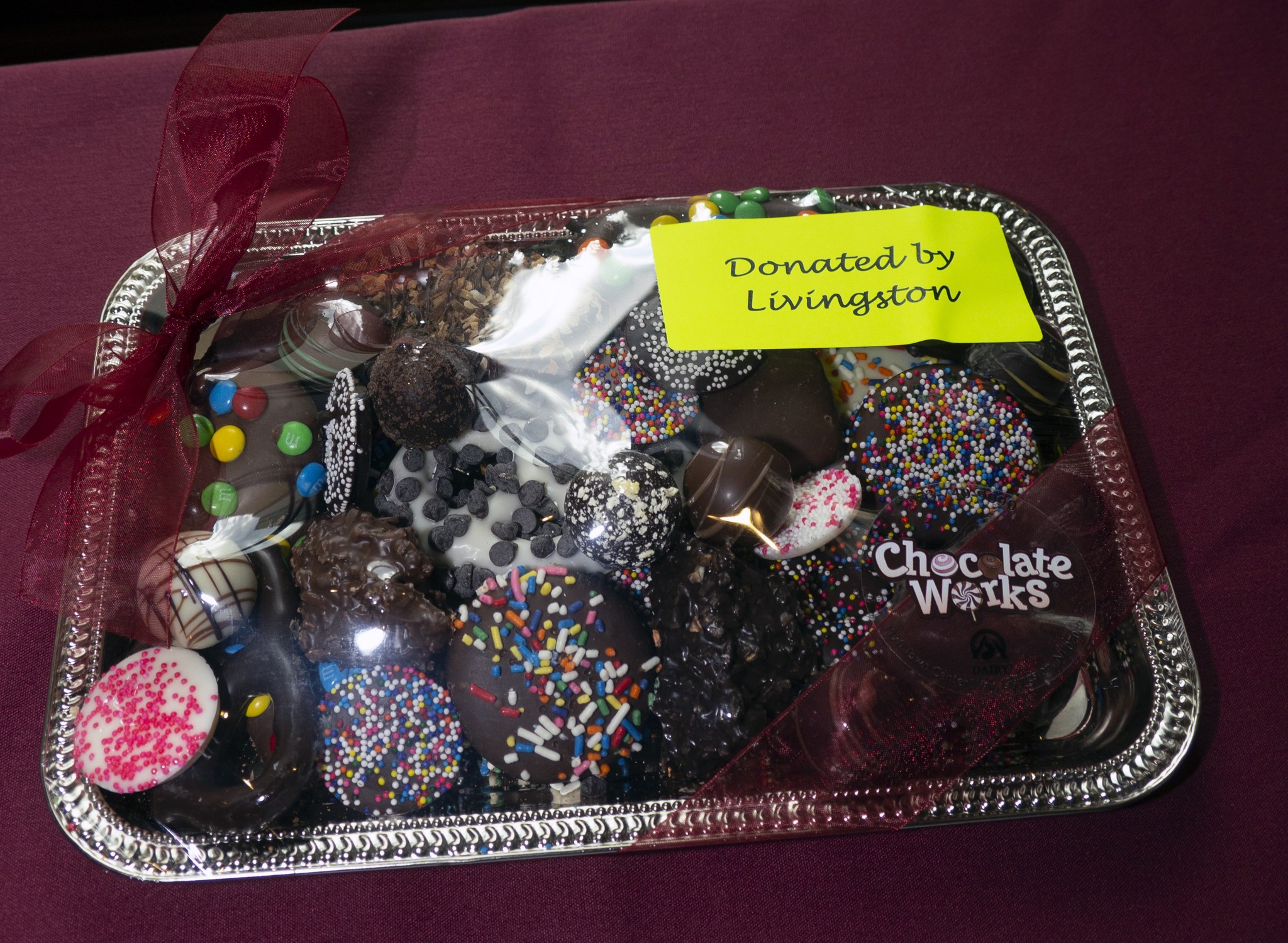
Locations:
[75,217,1046,817]
